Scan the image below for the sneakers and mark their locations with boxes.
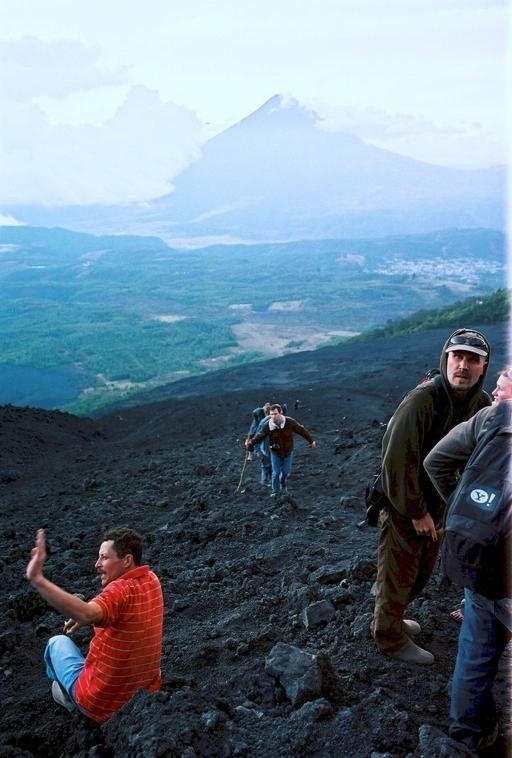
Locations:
[386,633,436,668]
[270,492,279,497]
[280,483,288,495]
[369,618,423,635]
[247,455,253,461]
[50,680,77,715]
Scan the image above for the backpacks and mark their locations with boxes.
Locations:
[438,398,512,602]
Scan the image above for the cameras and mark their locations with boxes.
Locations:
[270,444,280,450]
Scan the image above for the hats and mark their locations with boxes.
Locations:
[443,331,489,358]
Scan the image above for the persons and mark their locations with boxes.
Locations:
[26,528,162,725]
[449,370,512,622]
[423,399,512,752]
[246,401,316,497]
[370,328,491,667]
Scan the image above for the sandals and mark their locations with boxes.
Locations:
[449,607,465,624]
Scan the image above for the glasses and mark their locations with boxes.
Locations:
[446,336,486,351]
